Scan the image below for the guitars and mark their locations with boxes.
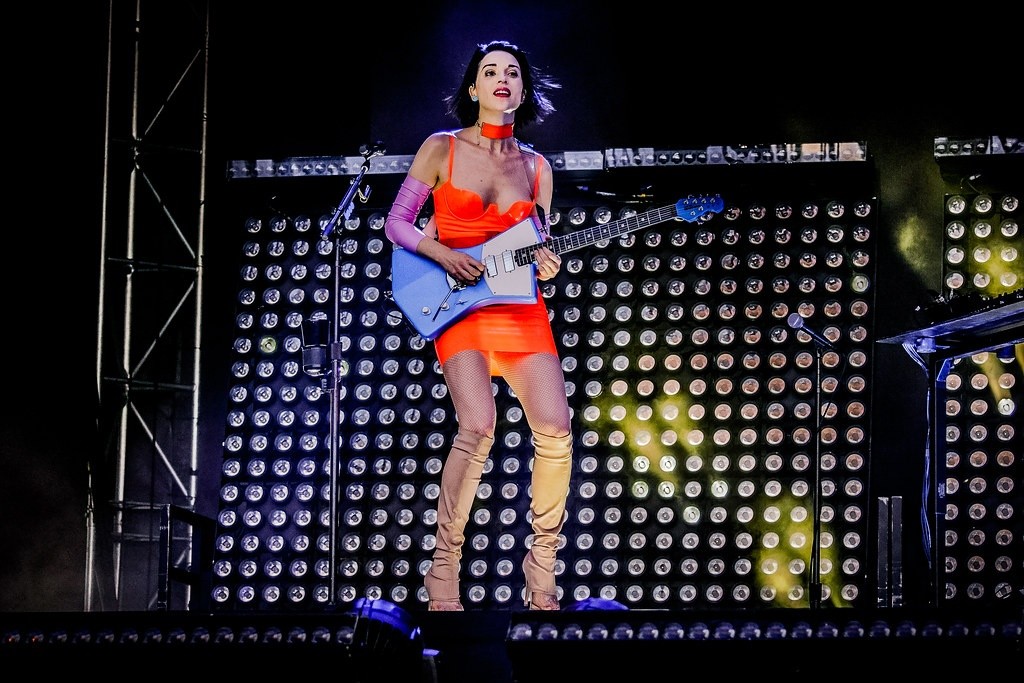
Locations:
[388,189,726,342]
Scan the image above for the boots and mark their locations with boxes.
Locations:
[424,429,495,611]
[522,430,573,610]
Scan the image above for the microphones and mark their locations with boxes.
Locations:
[360,141,386,160]
[787,313,835,351]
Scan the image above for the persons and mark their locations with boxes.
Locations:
[384,41,572,612]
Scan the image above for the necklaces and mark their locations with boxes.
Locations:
[474,121,515,145]
